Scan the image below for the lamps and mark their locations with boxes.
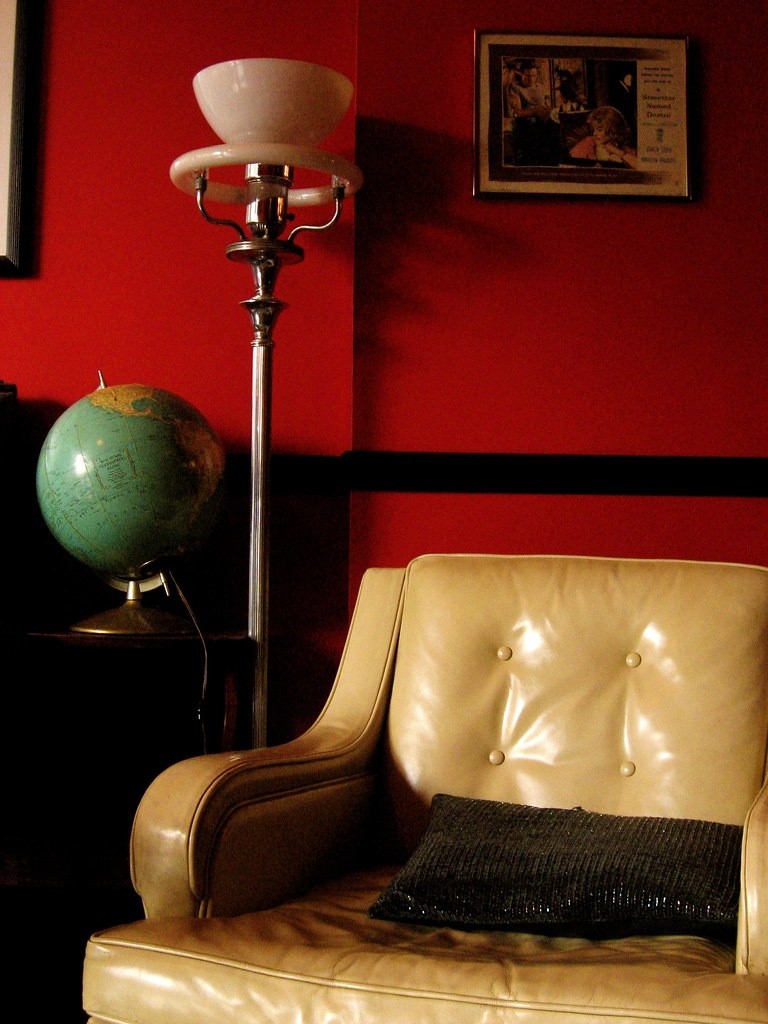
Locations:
[170,56,363,752]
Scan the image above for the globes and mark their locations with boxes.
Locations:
[33,364,228,640]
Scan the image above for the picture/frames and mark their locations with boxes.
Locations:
[473,28,705,203]
[0,0,54,280]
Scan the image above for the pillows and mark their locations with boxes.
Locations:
[365,792,741,951]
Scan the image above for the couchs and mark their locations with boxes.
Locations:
[81,553,765,1024]
